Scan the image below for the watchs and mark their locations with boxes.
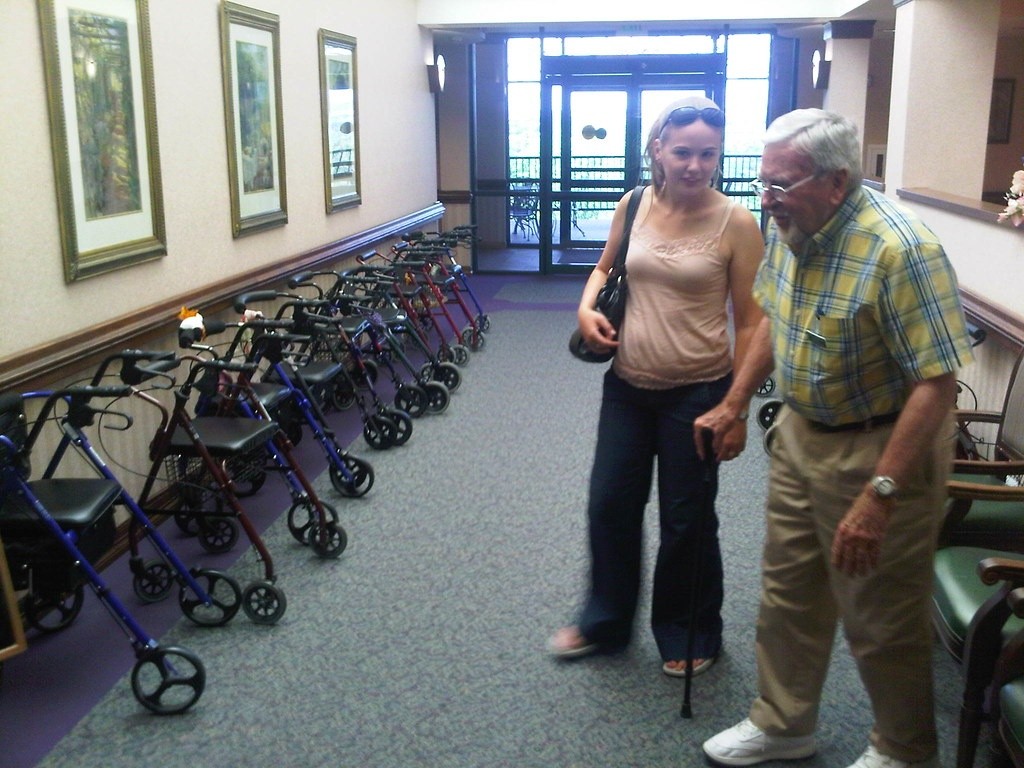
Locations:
[869,475,907,501]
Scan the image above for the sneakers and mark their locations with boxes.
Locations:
[702,717,818,765]
[846,745,908,768]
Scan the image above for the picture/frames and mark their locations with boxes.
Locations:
[318,28,362,214]
[221,0,288,239]
[36,0,167,285]
[986,77,1015,144]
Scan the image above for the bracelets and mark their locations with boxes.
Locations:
[738,409,750,422]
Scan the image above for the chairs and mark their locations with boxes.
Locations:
[927,346,1024,768]
[510,181,541,240]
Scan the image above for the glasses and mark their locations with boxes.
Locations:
[658,107,725,136]
[750,175,815,203]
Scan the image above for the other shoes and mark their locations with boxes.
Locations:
[662,652,720,675]
[545,625,599,658]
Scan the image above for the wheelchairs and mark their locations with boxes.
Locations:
[754,317,985,473]
[1,222,491,717]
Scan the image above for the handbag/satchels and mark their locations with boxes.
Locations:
[569,183,647,362]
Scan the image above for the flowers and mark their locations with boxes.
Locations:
[996,170,1024,226]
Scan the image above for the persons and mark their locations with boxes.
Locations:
[694,108,972,768]
[542,94,770,677]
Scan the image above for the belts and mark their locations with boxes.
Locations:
[814,413,898,432]
